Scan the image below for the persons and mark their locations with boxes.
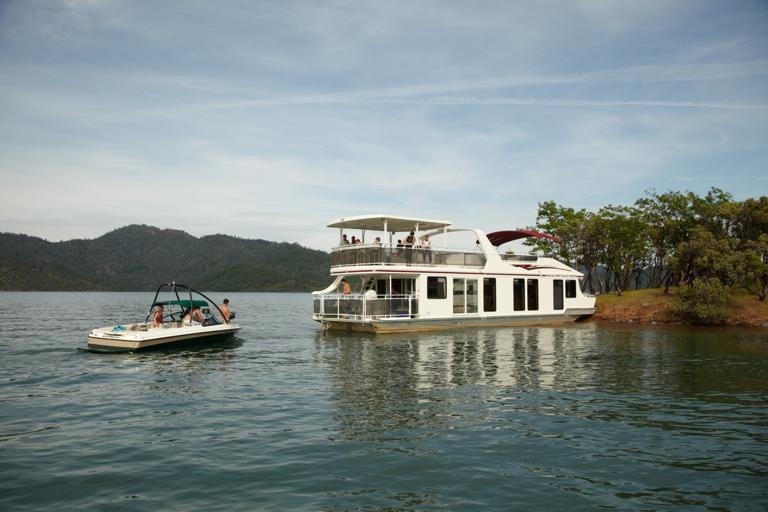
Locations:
[391,288,399,304]
[340,278,352,295]
[421,234,432,263]
[191,306,204,323]
[219,298,231,322]
[154,305,164,326]
[404,231,416,266]
[338,234,350,246]
[351,236,359,244]
[183,308,192,325]
[373,237,381,246]
[355,238,361,244]
[396,239,403,253]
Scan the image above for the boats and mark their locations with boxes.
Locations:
[311,215,597,332]
[88,283,240,353]
